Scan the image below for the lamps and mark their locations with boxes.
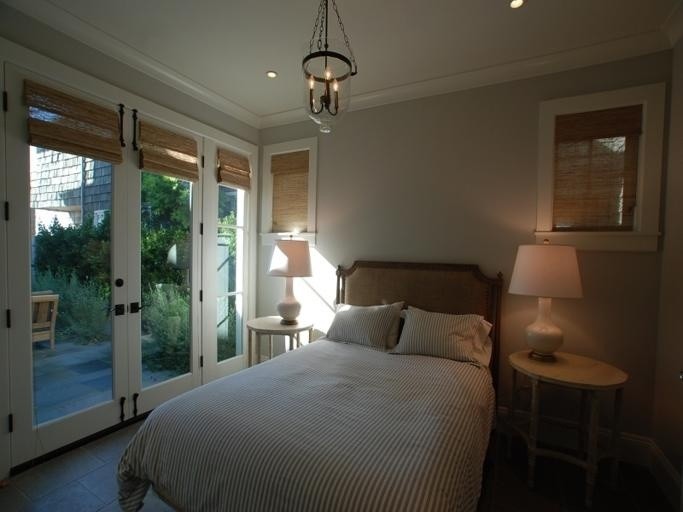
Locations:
[297,0,359,135]
[265,235,313,326]
[504,240,585,365]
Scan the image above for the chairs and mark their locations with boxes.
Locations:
[30,288,60,352]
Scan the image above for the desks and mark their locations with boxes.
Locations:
[505,345,629,510]
[245,315,316,370]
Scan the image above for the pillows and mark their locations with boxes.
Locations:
[323,300,493,369]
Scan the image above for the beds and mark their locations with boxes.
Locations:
[139,258,503,512]
